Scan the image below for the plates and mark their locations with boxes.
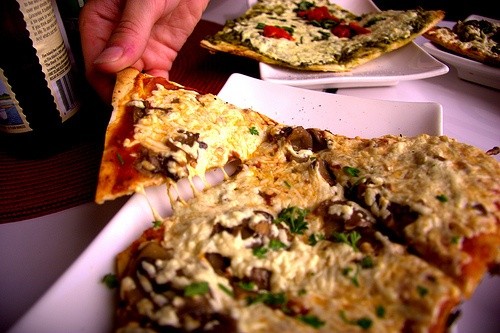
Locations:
[422,15,500,90]
[248,0,449,88]
[8,72,444,333]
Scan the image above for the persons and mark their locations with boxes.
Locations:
[79,0,210,105]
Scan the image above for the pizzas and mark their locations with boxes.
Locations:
[421,19,500,66]
[95,67,500,333]
[199,0,445,72]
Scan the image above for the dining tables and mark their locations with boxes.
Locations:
[1,19,261,224]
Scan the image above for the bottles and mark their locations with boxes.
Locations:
[0,0,83,162]
